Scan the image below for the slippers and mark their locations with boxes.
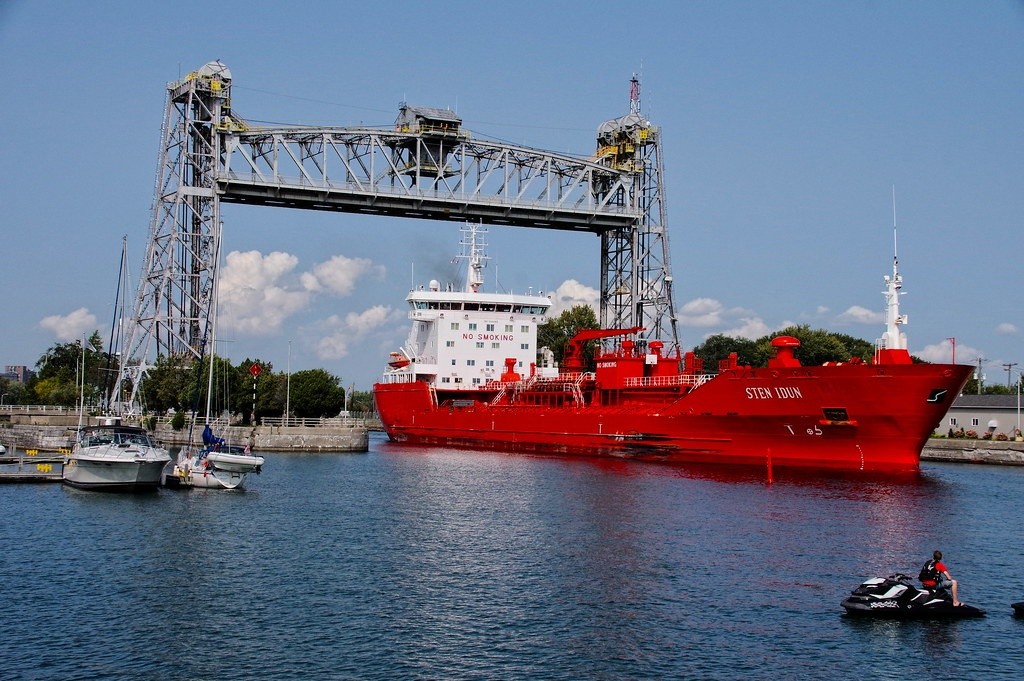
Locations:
[953,602,967,608]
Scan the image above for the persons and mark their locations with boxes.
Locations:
[244,444,251,456]
[918,550,965,607]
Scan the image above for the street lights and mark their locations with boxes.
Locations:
[287,339,291,427]
[96,352,121,406]
[1,393,10,405]
[345,386,351,425]
[76,352,82,405]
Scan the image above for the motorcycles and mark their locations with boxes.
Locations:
[840,571,987,618]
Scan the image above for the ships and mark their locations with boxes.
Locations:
[372,182,978,486]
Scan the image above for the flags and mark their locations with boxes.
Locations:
[82,335,97,352]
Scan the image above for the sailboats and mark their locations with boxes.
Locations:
[66,235,173,490]
[159,220,265,489]
